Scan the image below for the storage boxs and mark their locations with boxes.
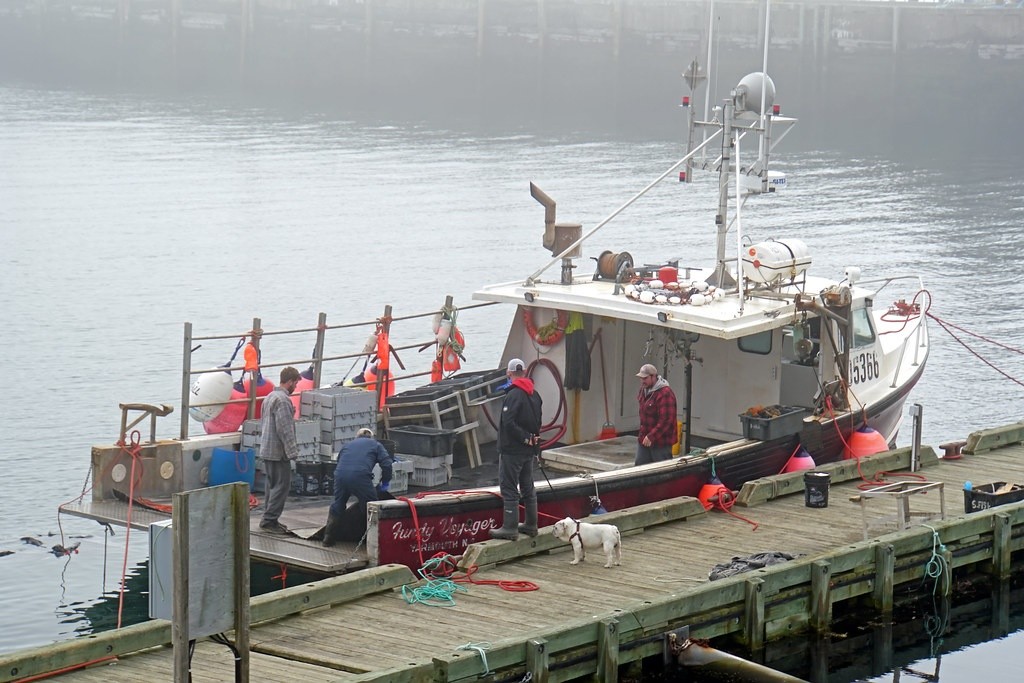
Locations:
[738,405,806,441]
[372,368,506,494]
[962,482,1024,513]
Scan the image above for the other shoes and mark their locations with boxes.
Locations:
[264,522,287,534]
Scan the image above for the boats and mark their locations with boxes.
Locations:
[59,2,930,569]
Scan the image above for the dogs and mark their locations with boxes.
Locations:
[552,517,622,568]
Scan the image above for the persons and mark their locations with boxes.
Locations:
[489,358,542,540]
[635,364,678,466]
[322,428,393,547]
[258,366,302,531]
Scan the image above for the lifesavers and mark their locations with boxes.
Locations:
[523,306,570,345]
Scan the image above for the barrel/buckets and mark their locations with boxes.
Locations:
[804,471,831,508]
[742,238,812,283]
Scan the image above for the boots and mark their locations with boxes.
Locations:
[518,497,538,536]
[322,509,339,547]
[488,500,520,542]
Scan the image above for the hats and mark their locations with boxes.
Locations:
[357,428,373,436]
[635,363,657,378]
[508,359,526,371]
